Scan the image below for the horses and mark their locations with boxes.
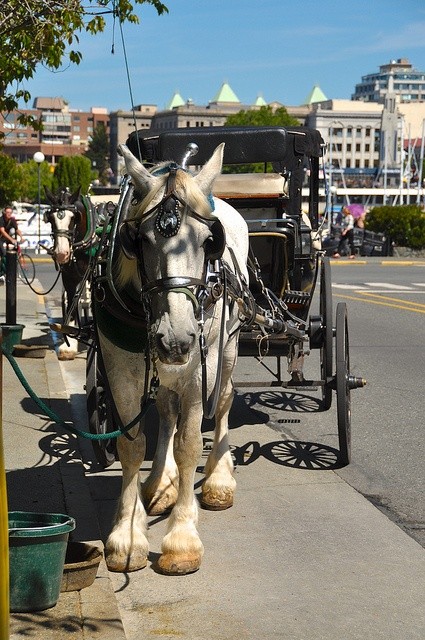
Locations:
[88,142,249,577]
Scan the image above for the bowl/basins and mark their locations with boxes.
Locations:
[59,542,103,592]
[13,344,49,359]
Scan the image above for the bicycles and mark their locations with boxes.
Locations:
[0,239,36,285]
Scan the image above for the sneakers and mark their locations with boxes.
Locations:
[332,253,340,258]
[349,254,355,259]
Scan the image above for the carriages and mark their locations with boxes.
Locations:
[83,124,367,576]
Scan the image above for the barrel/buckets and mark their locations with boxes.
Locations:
[8,511,76,611]
[0,323,25,357]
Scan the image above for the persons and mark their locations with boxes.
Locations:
[333,205,357,259]
[0,206,25,286]
